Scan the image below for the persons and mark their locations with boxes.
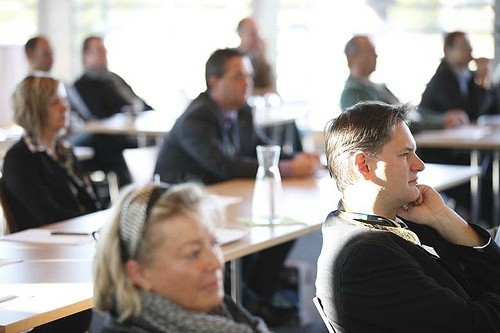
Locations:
[84,180,274,333]
[0,73,105,332]
[314,98,500,333]
[233,17,279,96]
[151,47,322,326]
[341,33,495,223]
[418,29,500,173]
[74,37,157,152]
[22,35,133,191]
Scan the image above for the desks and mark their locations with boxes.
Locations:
[415,121,500,230]
[80,102,329,132]
[0,165,480,333]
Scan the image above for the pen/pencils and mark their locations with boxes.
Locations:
[50,231,91,236]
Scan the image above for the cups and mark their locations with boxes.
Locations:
[92,227,110,242]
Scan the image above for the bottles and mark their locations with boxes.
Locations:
[252,145,285,225]
